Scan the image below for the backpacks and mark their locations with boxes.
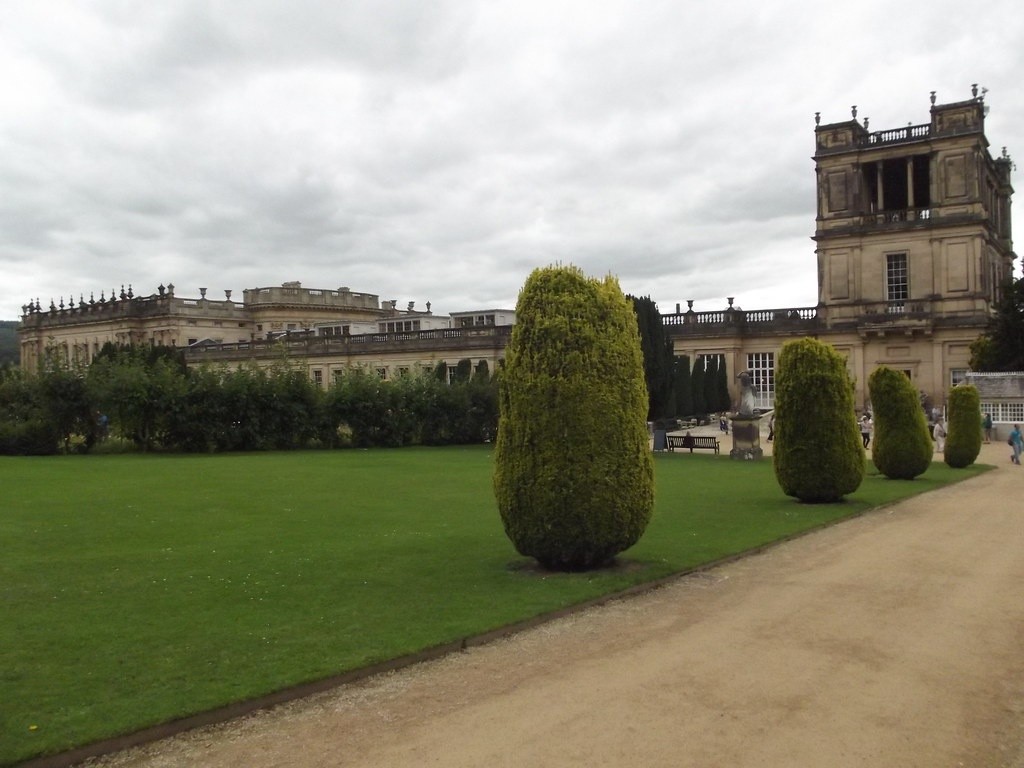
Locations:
[1007,435,1013,446]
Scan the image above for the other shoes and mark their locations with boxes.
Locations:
[983,441,990,444]
[1011,455,1015,462]
[1016,460,1020,464]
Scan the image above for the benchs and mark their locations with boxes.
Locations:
[667,436,720,455]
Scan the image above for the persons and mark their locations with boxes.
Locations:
[719,412,729,434]
[860,417,873,450]
[931,405,947,452]
[766,415,775,443]
[1009,424,1024,465]
[981,413,993,444]
